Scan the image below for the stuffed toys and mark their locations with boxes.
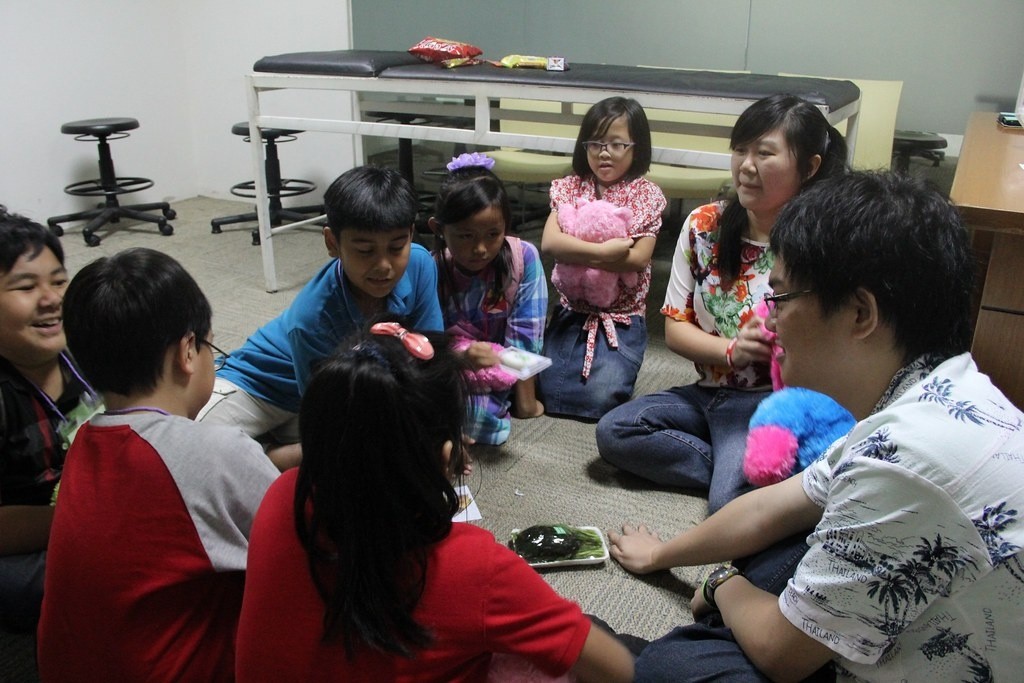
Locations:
[743,385,856,486]
[551,199,637,306]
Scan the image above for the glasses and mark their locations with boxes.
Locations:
[765,281,884,317]
[582,141,638,156]
[179,332,230,372]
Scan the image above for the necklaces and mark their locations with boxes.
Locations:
[105,407,172,417]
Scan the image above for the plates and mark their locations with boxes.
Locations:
[505,526,609,569]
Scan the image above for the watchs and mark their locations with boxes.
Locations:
[706,564,744,607]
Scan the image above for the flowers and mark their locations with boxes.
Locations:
[445,151,495,172]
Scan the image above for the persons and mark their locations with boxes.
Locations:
[595,92,848,514]
[529,97,666,418]
[429,151,548,448]
[608,169,1024,682]
[194,163,477,479]
[235,321,638,683]
[0,216,102,631]
[37,247,283,683]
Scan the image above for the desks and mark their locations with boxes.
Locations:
[946,112,1024,412]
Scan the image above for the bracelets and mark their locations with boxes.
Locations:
[726,338,742,373]
[702,578,715,610]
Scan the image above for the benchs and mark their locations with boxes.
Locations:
[246,45,856,294]
[482,65,905,248]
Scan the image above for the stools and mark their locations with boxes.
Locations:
[886,129,947,182]
[364,99,438,203]
[42,112,177,248]
[209,116,328,245]
